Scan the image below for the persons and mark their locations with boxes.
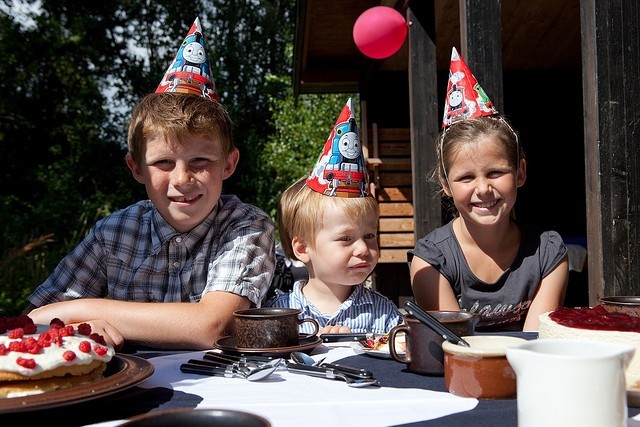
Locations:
[260,174,408,336]
[410,115,569,333]
[24,91,274,349]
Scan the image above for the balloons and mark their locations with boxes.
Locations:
[353,6,409,59]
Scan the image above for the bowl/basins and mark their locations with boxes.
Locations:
[537,308,640,391]
[440,337,525,400]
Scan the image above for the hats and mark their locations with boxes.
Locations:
[440,46,500,128]
[153,17,218,103]
[306,98,371,198]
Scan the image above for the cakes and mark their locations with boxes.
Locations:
[1,316,117,400]
[537,303,637,406]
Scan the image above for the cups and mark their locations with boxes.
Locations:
[388,311,472,378]
[232,308,320,345]
[505,341,637,427]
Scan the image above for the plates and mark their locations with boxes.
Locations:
[117,408,272,427]
[212,332,321,358]
[354,338,411,360]
[0,351,156,417]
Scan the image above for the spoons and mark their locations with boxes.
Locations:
[319,332,391,342]
[402,299,475,350]
[180,352,282,382]
[287,365,379,389]
[290,351,374,379]
